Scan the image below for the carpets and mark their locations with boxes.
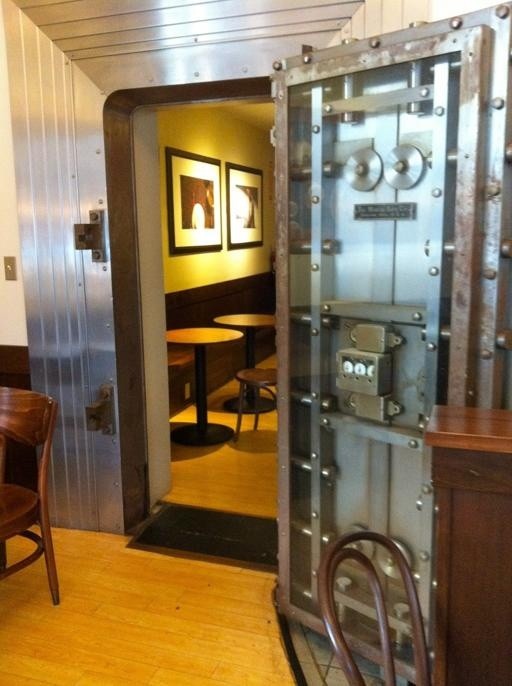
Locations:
[125,500,279,574]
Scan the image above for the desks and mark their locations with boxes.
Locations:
[166,312,280,447]
[422,400,511,684]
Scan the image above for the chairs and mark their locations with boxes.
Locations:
[319,526,435,684]
[0,387,64,608]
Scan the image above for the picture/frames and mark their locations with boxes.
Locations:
[164,140,262,258]
